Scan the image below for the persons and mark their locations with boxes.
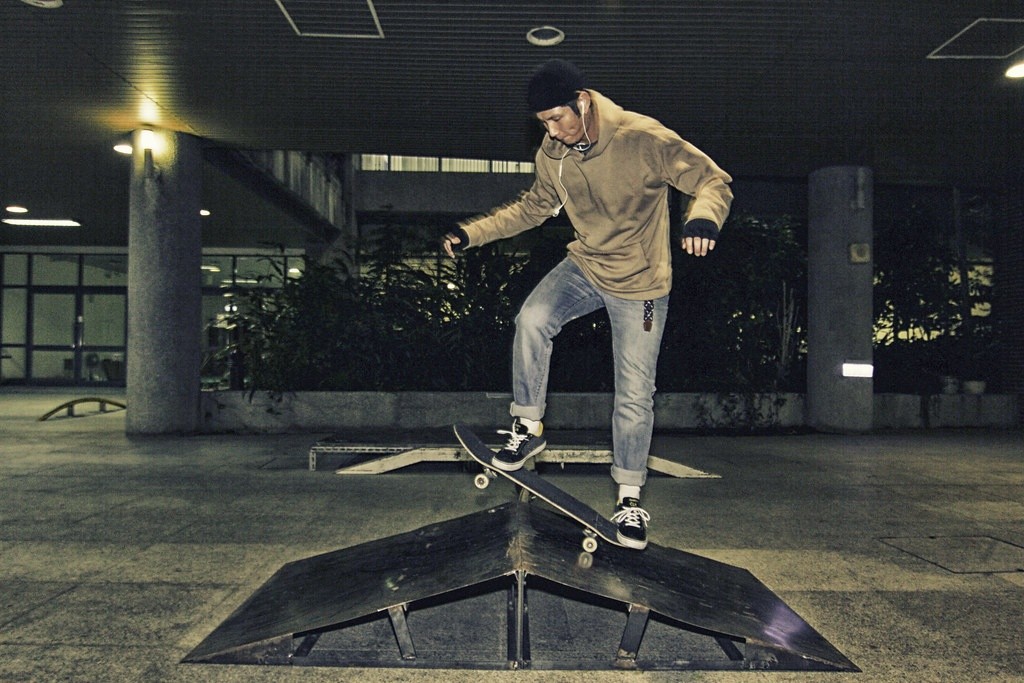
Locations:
[443,59,734,549]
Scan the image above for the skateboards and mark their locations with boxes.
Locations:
[452,421,644,555]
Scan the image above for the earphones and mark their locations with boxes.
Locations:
[555,206,562,216]
[580,101,585,114]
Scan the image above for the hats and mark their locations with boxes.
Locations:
[525,58,586,114]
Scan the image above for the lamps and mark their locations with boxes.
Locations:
[849,166,867,211]
[113,134,132,155]
[143,148,160,181]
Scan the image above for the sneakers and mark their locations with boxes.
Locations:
[491,419,547,471]
[614,496,652,549]
